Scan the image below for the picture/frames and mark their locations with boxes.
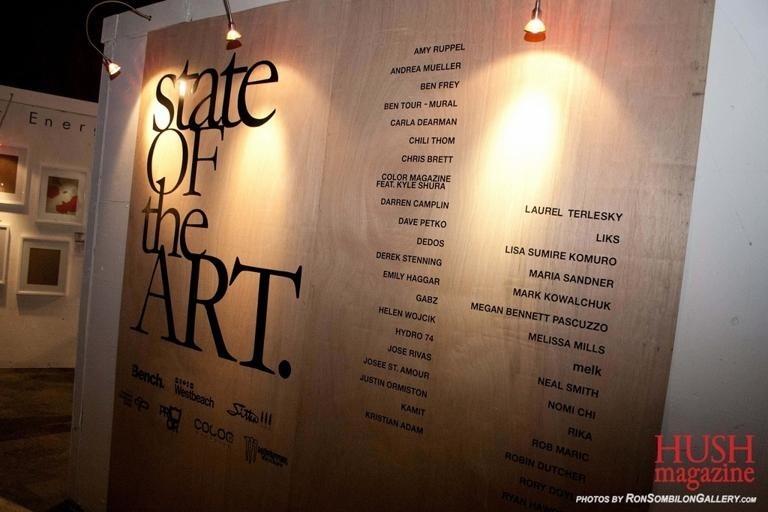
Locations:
[0,143,92,298]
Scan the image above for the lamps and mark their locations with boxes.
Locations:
[221,1,244,45]
[84,0,153,80]
[524,1,545,37]
